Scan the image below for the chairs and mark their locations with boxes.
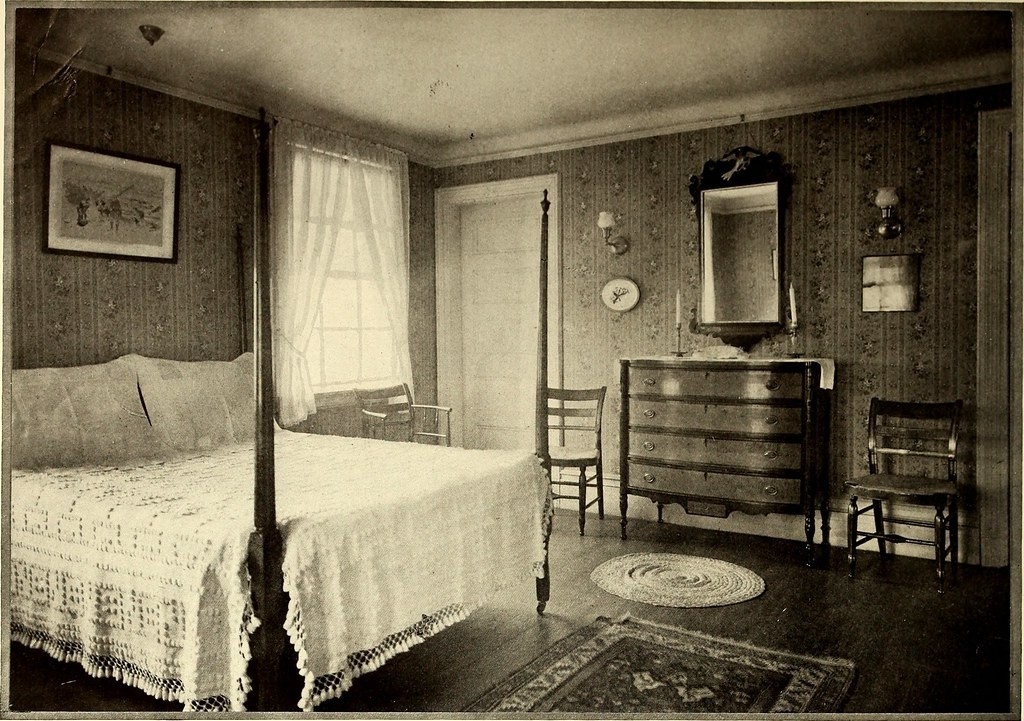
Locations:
[548,385,609,539]
[353,380,456,444]
[842,396,966,597]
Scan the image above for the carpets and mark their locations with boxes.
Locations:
[460,612,859,712]
[587,550,767,608]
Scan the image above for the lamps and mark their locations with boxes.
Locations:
[596,211,630,255]
[873,184,906,241]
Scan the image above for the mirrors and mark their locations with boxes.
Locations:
[687,144,792,351]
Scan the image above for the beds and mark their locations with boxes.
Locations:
[11,108,555,712]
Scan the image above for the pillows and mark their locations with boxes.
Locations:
[13,353,184,469]
[137,350,299,451]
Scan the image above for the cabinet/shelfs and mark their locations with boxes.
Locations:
[617,355,838,571]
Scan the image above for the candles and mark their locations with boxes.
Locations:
[674,288,683,324]
[789,281,798,326]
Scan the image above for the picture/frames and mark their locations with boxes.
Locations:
[860,253,922,314]
[42,139,181,266]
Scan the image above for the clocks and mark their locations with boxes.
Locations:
[599,275,640,313]
